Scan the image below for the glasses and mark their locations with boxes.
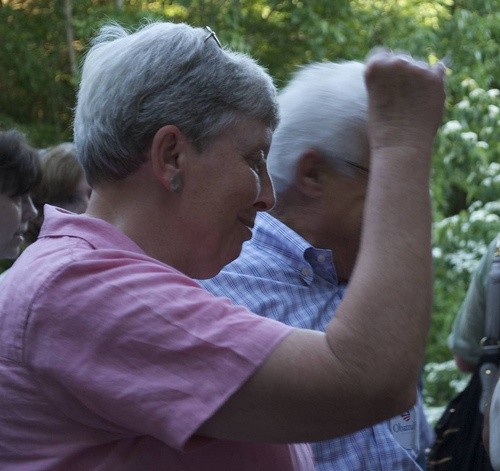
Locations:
[138,26,222,113]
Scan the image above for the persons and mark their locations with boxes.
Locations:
[23,142,92,245]
[0,19,447,471]
[0,131,43,261]
[449,232,500,471]
[192,60,436,471]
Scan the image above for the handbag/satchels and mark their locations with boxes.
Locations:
[424,352,500,471]
